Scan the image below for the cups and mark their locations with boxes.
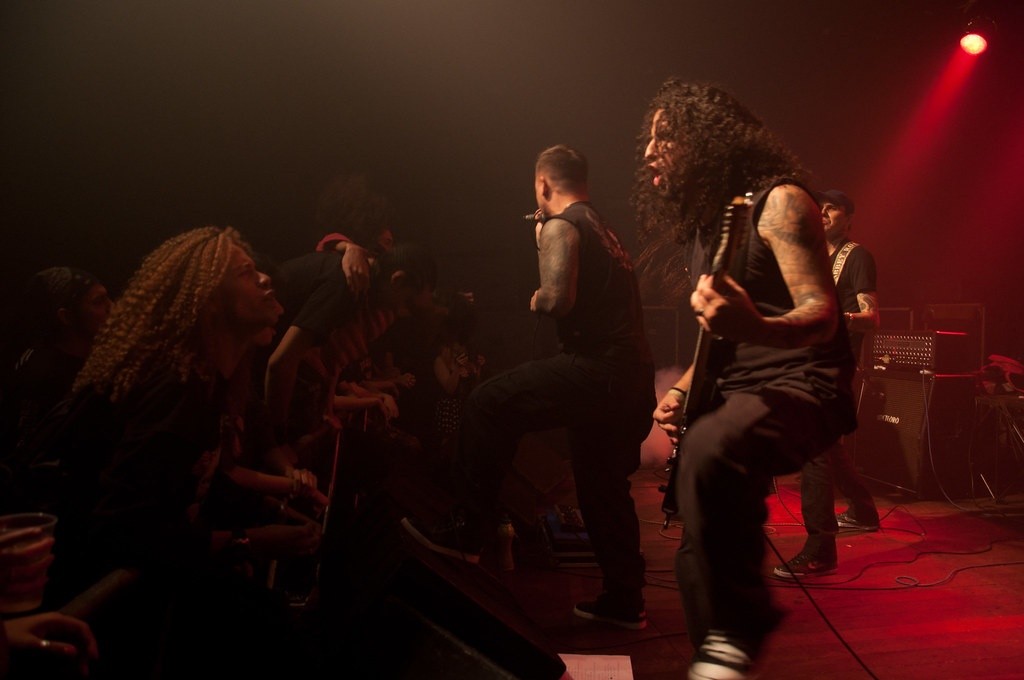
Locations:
[0,513,58,613]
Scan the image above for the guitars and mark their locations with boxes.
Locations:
[655,191,752,521]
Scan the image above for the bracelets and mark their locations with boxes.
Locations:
[670,387,686,397]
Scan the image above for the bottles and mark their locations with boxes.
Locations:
[495,517,516,571]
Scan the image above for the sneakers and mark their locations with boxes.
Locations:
[836,510,881,531]
[400,510,484,565]
[685,628,755,680]
[573,595,647,630]
[774,551,838,579]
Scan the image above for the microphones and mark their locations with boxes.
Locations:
[523,213,548,223]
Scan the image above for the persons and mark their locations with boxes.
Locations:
[0,174,486,680]
[400,146,656,631]
[774,189,880,580]
[631,76,856,680]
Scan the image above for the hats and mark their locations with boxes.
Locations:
[814,189,855,214]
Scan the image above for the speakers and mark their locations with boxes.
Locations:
[282,457,566,680]
[850,367,999,501]
